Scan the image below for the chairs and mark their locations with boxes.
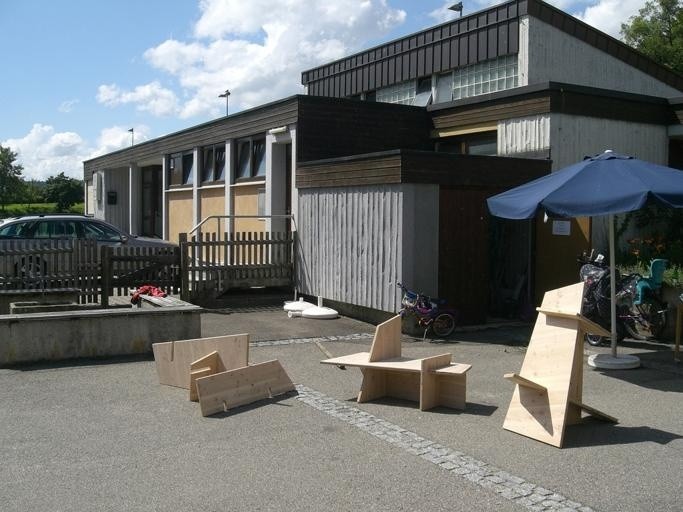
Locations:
[633,259,664,305]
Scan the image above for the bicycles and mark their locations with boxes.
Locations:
[398,283,456,340]
[576,251,682,349]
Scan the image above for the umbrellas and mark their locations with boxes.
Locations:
[486,150,682,357]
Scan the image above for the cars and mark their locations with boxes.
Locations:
[0,215,191,290]
